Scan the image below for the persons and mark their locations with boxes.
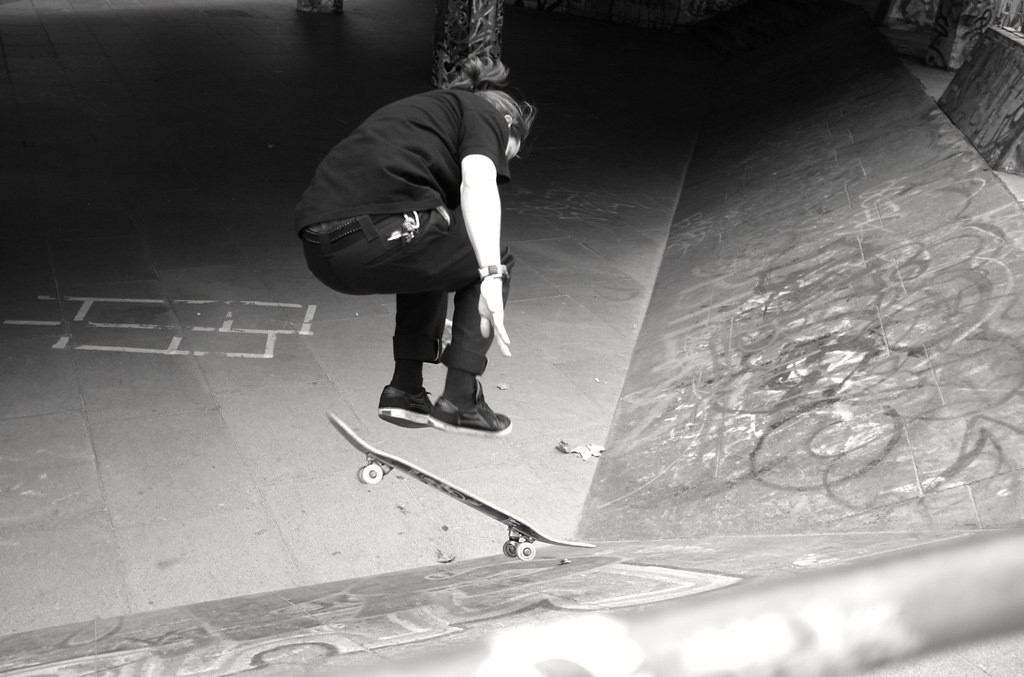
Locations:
[292,51,538,438]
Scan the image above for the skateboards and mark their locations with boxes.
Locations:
[326,410,597,563]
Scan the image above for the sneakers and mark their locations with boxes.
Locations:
[378,385,433,429]
[427,380,512,436]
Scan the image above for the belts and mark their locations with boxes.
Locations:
[301,214,392,244]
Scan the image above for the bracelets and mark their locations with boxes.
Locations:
[478,264,510,281]
